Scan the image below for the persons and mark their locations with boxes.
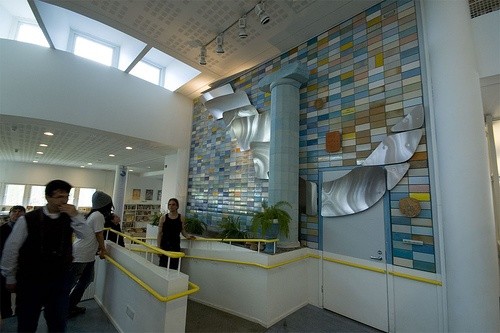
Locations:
[156,198,196,269]
[0,180,123,333]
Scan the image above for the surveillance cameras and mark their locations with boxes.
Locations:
[120,171,126,176]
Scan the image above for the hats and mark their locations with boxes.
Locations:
[91,190,112,210]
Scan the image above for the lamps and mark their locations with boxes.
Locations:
[253,3,271,26]
[215,33,225,54]
[237,16,248,40]
[199,45,208,67]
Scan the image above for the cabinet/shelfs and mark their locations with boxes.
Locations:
[124,203,161,220]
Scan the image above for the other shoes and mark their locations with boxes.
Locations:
[69,306,86,317]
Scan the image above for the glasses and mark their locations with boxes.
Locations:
[48,195,69,199]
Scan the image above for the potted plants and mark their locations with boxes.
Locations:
[251,200,295,253]
